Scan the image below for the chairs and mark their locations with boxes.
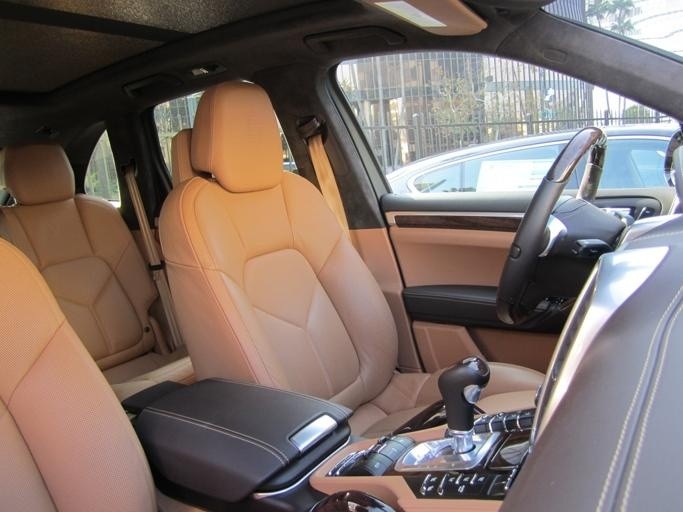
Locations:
[161,77,545,438]
[2,135,194,407]
[0,237,160,510]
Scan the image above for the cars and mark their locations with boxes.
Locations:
[383,122,682,197]
